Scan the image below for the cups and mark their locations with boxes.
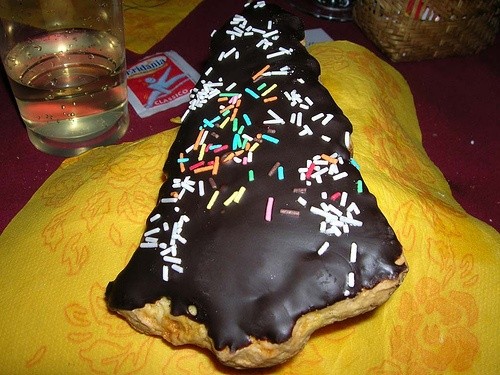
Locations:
[289,0,359,22]
[0,0,131,158]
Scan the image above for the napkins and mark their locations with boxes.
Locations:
[0,41,500,374]
[0,1,203,55]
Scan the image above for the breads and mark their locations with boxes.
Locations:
[103,0,408,370]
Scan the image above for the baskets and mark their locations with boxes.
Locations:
[352,0,500,63]
